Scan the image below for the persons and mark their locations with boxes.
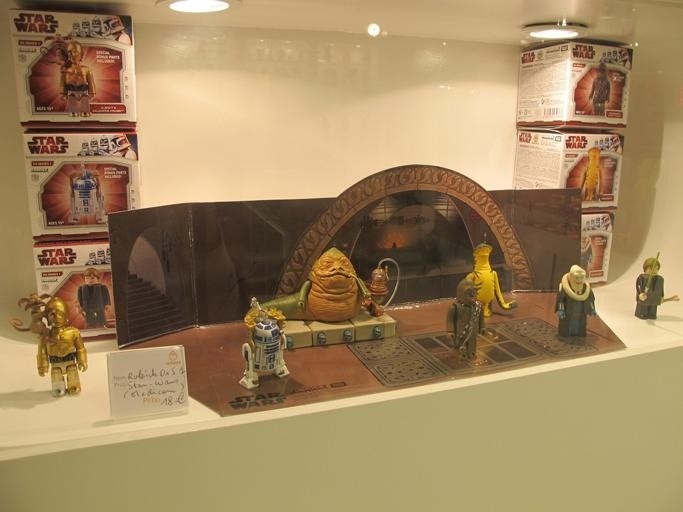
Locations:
[552,264,595,341]
[635,250,663,321]
[9,291,87,399]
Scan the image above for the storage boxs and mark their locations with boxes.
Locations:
[20,130,139,241]
[29,244,118,342]
[8,7,140,127]
[514,39,634,285]
